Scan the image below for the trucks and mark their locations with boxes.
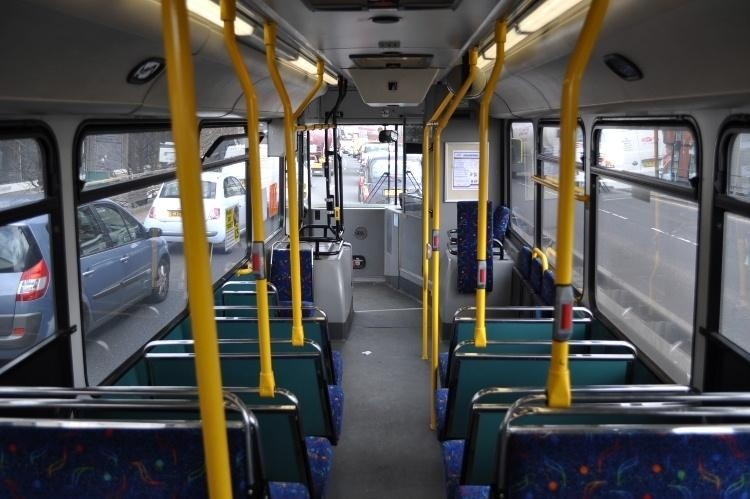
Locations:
[225,145,311,221]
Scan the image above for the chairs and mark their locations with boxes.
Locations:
[447,206,510,260]
[268,240,315,317]
[222,280,281,318]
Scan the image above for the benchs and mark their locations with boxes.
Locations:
[1,386,334,499]
[437,305,638,440]
[145,308,346,446]
[440,385,750,498]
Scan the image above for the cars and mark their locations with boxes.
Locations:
[307,127,422,203]
[0,190,172,361]
[142,170,246,254]
